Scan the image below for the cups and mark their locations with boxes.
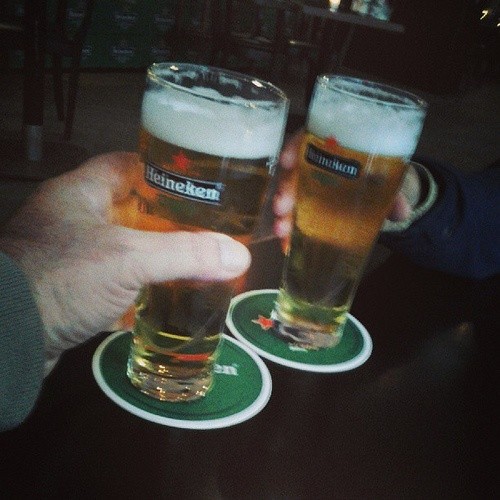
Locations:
[270,74,429,347]
[125,62,290,402]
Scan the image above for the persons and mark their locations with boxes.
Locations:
[272,125,500,280]
[0,152,253,433]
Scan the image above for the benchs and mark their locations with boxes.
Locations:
[179,22,318,62]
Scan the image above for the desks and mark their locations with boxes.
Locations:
[254,0,408,132]
